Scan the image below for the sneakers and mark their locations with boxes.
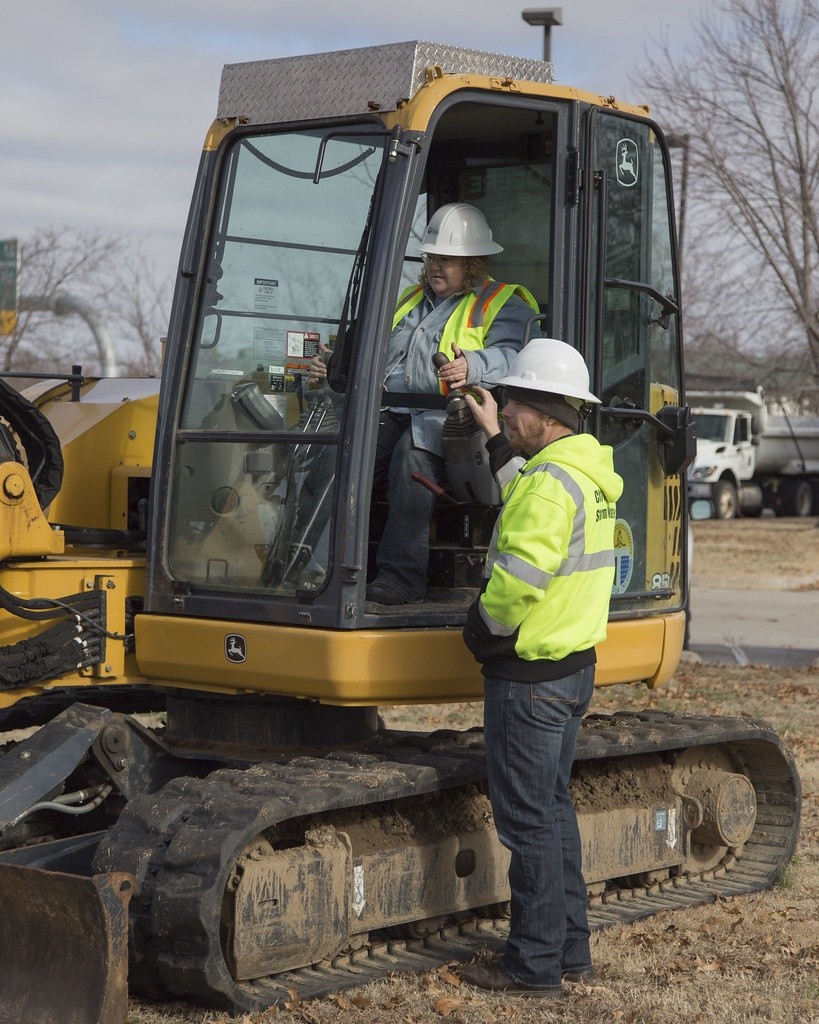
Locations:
[366,582,408,606]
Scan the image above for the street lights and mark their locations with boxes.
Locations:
[522,7,560,62]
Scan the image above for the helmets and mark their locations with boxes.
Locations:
[494,338,602,404]
[413,202,504,257]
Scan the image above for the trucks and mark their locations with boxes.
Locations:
[686,391,817,519]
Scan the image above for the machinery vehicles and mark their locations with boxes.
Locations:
[2,40,802,1022]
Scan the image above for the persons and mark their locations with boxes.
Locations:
[275,203,543,604]
[460,338,623,992]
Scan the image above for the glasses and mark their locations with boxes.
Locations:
[420,252,470,268]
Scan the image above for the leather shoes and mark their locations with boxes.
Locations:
[447,962,562,1000]
